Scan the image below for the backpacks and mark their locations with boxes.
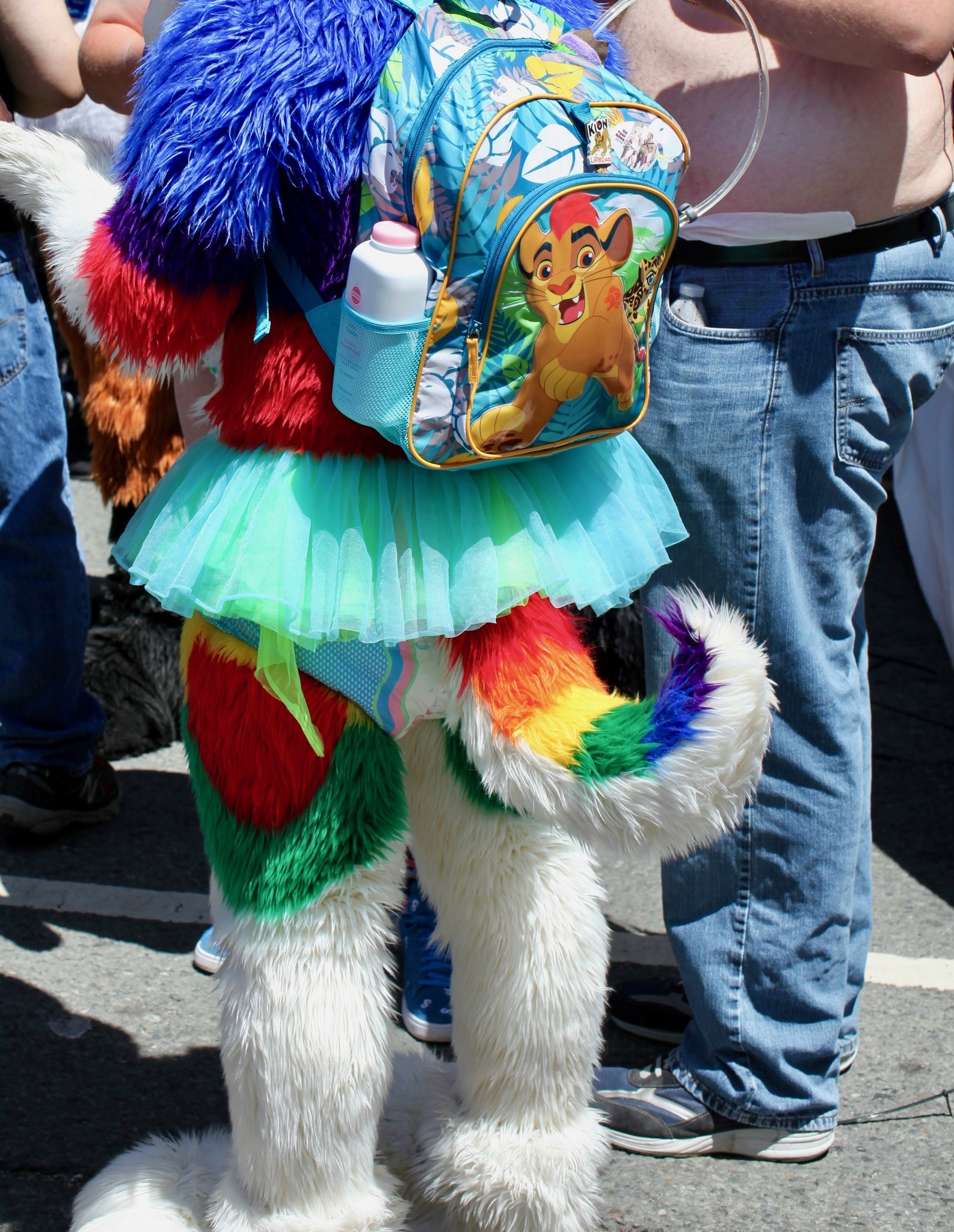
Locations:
[253,0,691,472]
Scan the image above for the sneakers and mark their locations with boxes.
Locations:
[194,926,230,974]
[388,857,453,1042]
[587,1051,835,1164]
[607,980,859,1074]
[0,762,120,834]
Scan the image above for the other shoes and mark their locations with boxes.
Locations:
[69,450,91,474]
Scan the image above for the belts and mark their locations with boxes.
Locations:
[672,196,954,269]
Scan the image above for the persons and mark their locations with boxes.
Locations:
[0,0,117,816]
[77,0,954,1158]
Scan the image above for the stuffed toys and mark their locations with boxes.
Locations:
[38,232,186,764]
[0,0,780,1232]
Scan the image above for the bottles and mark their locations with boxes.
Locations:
[345,219,430,324]
[670,282,709,328]
